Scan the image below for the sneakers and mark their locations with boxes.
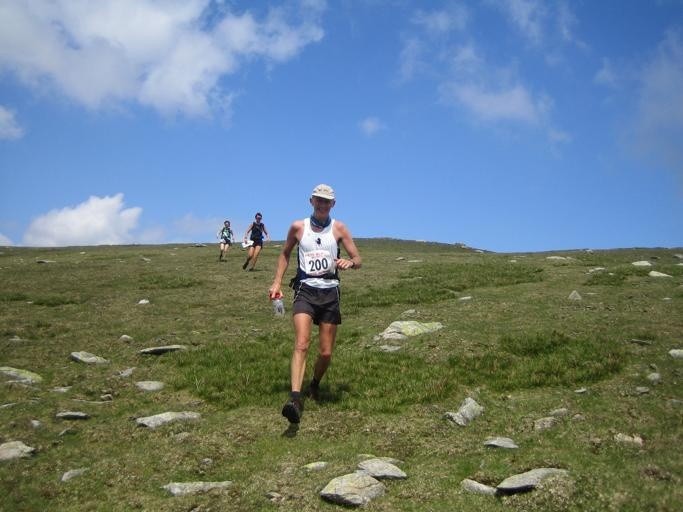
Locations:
[282,399,300,423]
[311,381,319,400]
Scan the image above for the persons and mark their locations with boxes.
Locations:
[264,183,363,427]
[215,221,234,263]
[242,212,267,269]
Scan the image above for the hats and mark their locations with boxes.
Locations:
[312,185,334,200]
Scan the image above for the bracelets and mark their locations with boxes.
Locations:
[348,258,355,269]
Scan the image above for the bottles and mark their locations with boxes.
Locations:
[271,293,285,320]
